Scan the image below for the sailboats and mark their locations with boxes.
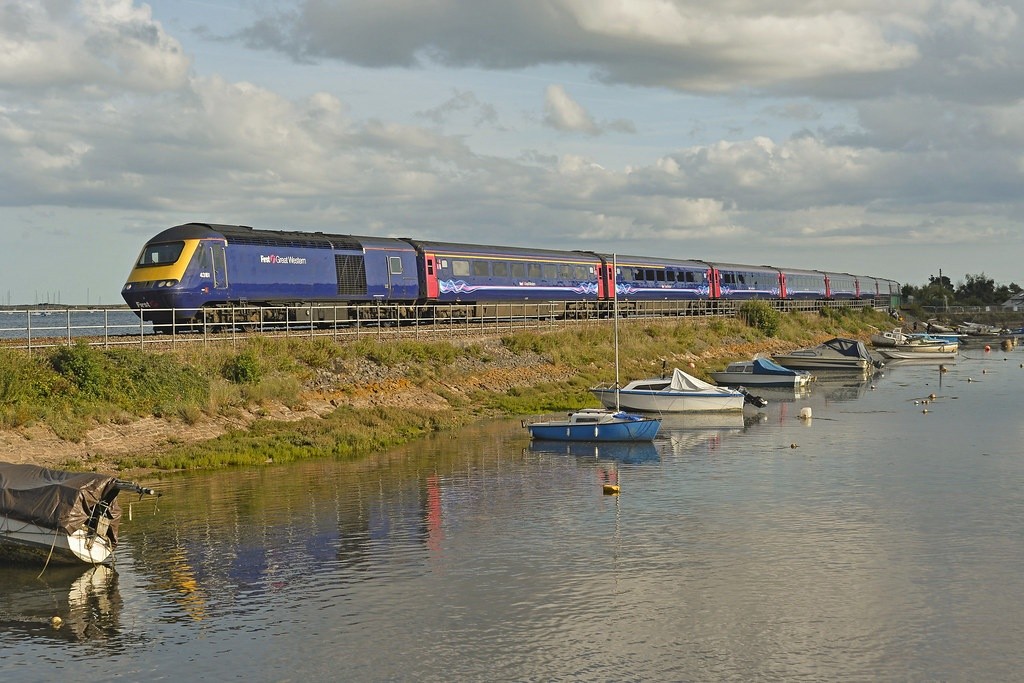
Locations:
[520,439,663,600]
[521,251,663,442]
[1,289,101,316]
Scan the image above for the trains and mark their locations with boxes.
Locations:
[121,222,903,333]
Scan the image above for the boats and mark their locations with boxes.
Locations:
[770,337,886,369]
[707,357,812,388]
[0,462,155,567]
[589,367,768,414]
[739,387,811,402]
[640,413,767,454]
[876,336,958,358]
[0,565,131,660]
[907,319,1024,345]
[808,370,872,401]
[870,328,912,346]
